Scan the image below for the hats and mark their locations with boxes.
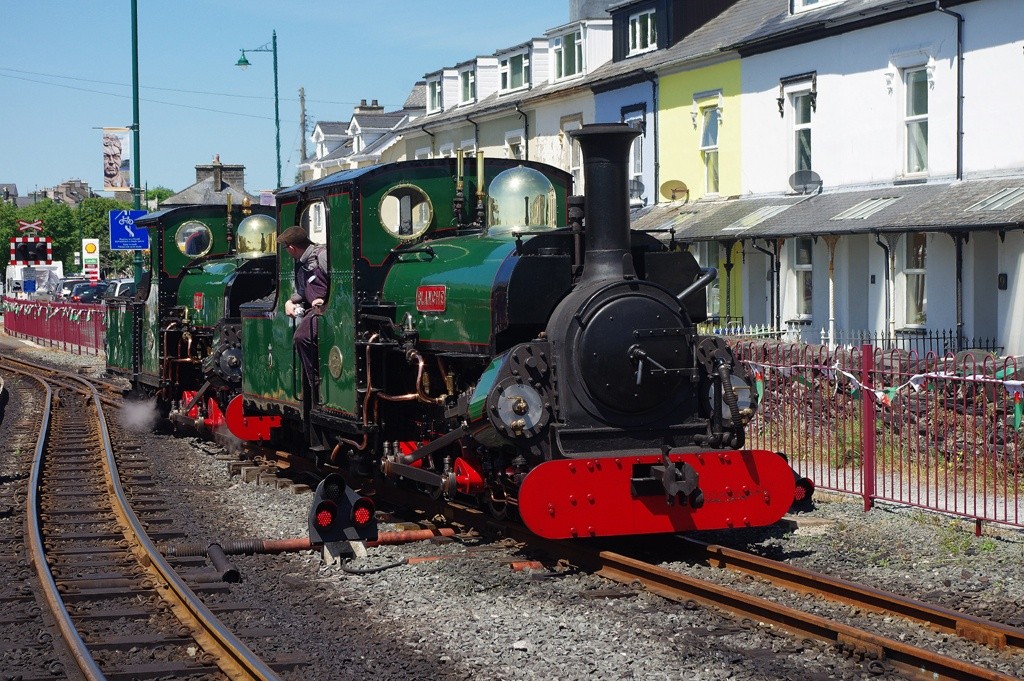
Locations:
[276,226,308,246]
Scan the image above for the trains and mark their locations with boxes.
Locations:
[99,121,818,547]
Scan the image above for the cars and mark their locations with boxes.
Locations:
[46,276,135,306]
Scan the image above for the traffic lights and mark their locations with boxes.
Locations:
[15,241,47,261]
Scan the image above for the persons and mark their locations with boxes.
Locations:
[277,227,327,388]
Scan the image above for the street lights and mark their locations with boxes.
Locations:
[69,190,83,272]
[234,28,282,189]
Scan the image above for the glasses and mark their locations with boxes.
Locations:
[282,246,295,250]
